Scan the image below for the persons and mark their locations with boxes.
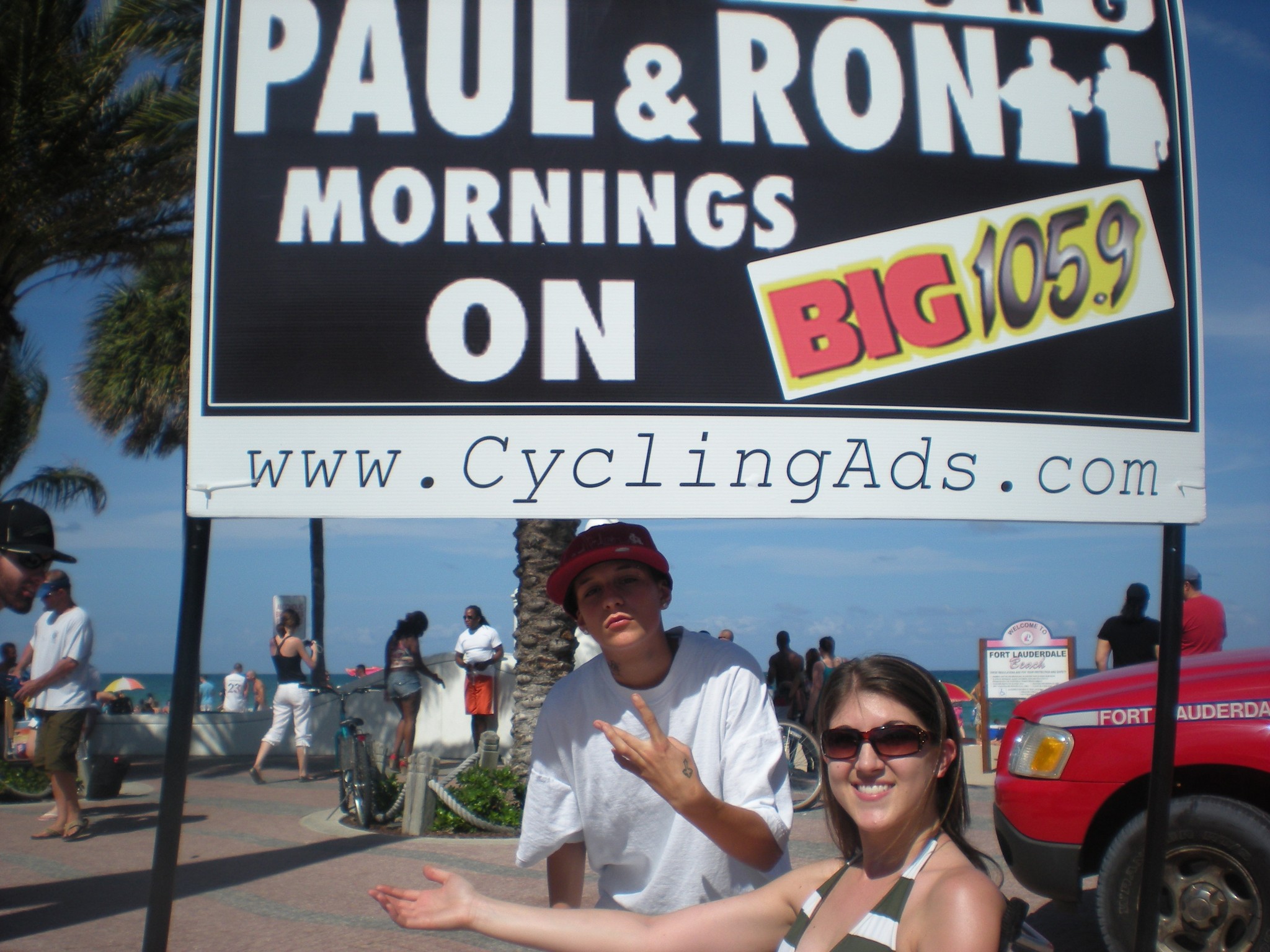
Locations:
[0,499,367,841]
[699,629,1004,770]
[454,605,504,752]
[366,654,1006,952]
[514,521,794,951]
[384,610,447,775]
[1095,564,1228,672]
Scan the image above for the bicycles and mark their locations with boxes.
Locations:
[311,682,388,830]
[775,719,825,813]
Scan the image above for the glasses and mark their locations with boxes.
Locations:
[18,552,52,571]
[463,615,474,620]
[822,725,933,760]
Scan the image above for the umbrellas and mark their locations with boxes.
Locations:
[938,680,973,703]
[103,676,145,693]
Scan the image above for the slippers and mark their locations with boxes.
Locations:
[30,828,59,839]
[63,817,89,841]
[38,812,59,823]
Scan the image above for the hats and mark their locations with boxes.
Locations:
[36,575,70,598]
[1182,564,1203,581]
[546,523,669,621]
[0,498,78,563]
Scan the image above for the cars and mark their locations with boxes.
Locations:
[993,645,1270,952]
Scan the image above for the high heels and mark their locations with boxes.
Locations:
[400,761,410,776]
[389,755,399,770]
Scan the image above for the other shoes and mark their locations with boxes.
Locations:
[250,768,266,785]
[299,776,317,784]
